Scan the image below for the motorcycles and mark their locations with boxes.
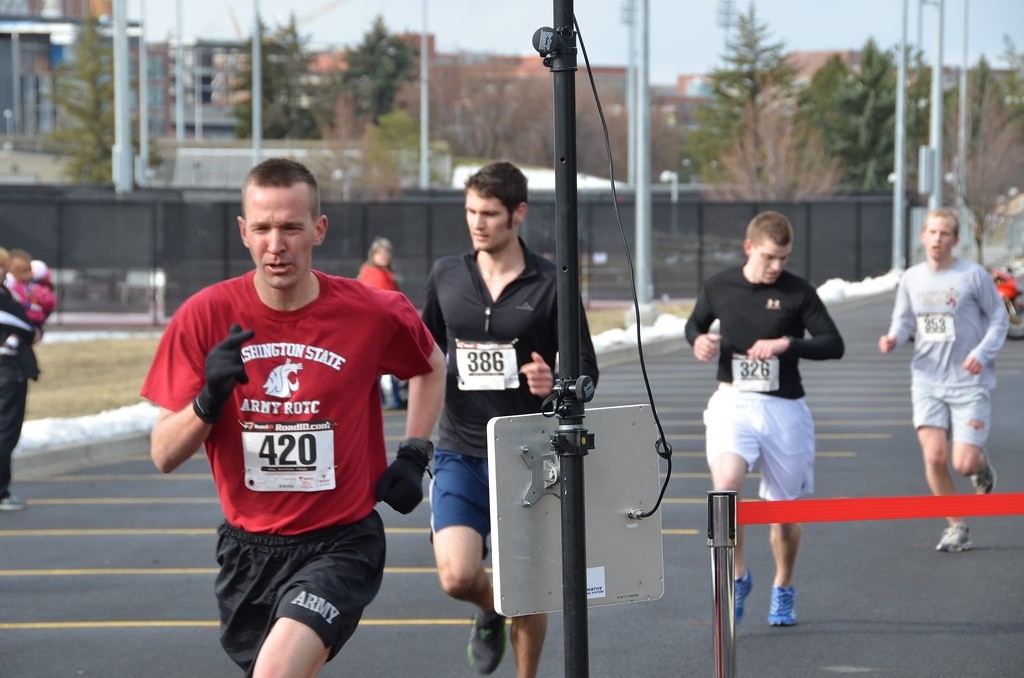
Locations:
[989,267,1024,339]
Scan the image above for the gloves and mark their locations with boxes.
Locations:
[192,324,254,424]
[375,446,433,516]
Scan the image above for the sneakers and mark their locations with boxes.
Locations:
[969,447,997,494]
[935,524,974,552]
[767,584,798,625]
[466,609,507,675]
[735,569,752,623]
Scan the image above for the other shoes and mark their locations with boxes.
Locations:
[0,495,27,510]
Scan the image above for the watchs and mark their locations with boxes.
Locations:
[398,435,434,460]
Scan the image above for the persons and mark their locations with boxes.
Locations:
[421,162,599,678]
[0,248,57,512]
[881,207,1010,553]
[136,158,444,678]
[356,236,407,409]
[684,210,846,627]
